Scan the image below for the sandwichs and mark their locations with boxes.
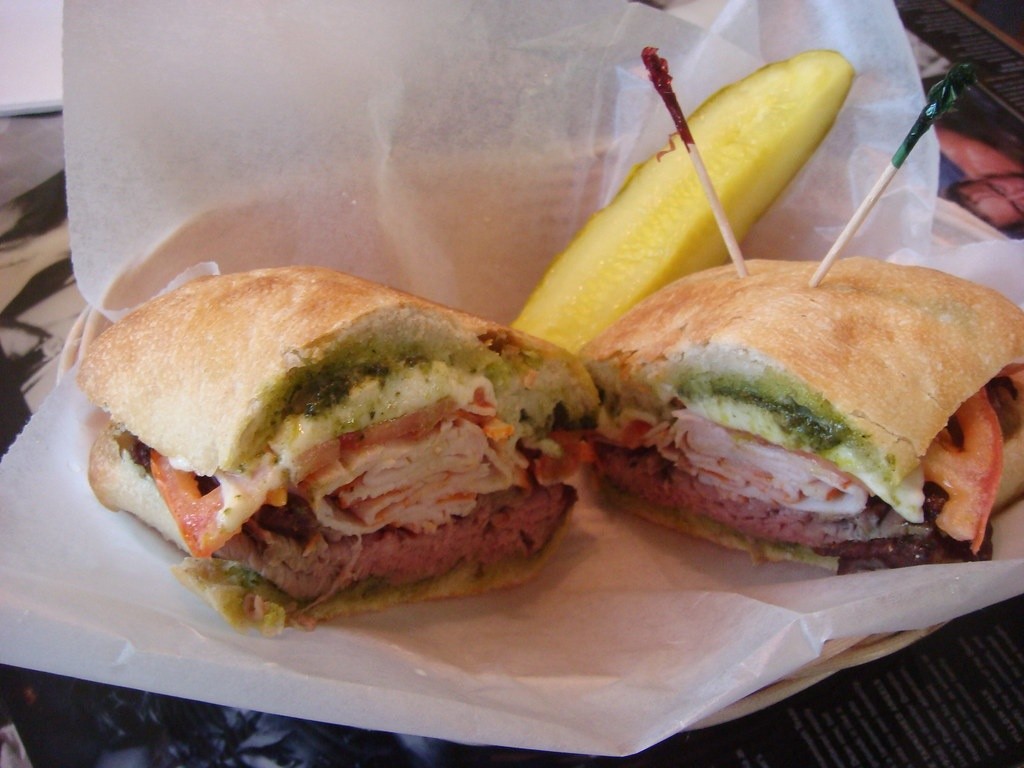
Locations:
[564,258,1024,571]
[75,263,618,638]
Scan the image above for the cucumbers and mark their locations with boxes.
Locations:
[508,51,853,348]
